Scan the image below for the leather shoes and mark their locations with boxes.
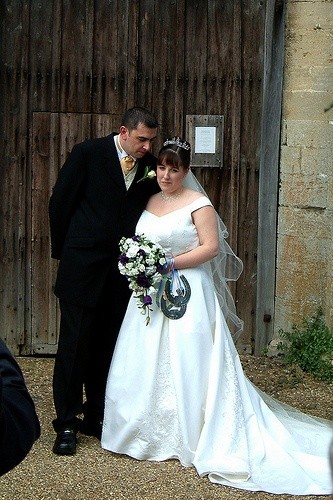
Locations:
[52,429,77,455]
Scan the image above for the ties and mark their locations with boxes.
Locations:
[121,157,134,176]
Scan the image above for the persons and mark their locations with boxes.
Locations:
[1,336,42,477]
[48,108,161,455]
[101,136,220,479]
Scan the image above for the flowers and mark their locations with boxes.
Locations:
[118,232,167,326]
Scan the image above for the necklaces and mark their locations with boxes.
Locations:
[161,185,184,202]
